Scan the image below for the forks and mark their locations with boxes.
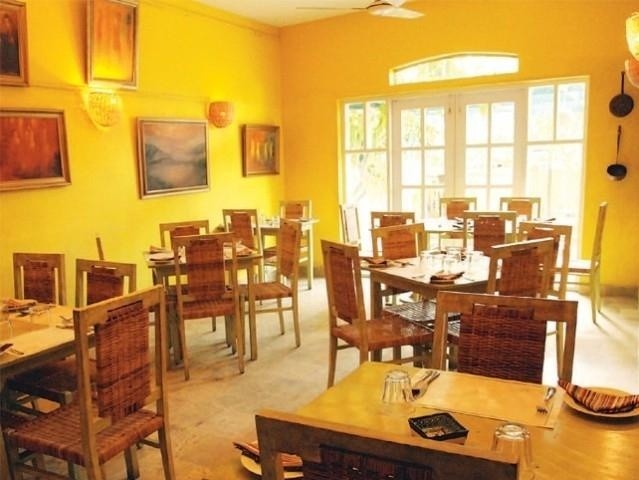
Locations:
[536,386,557,416]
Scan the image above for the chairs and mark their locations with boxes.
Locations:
[3,258,137,418]
[437,197,478,220]
[446,237,555,373]
[547,200,608,324]
[253,409,520,479]
[516,222,573,381]
[462,210,517,251]
[2,283,176,480]
[11,250,68,305]
[317,236,436,389]
[368,220,461,372]
[371,211,417,228]
[165,229,245,383]
[262,198,315,291]
[158,219,211,248]
[426,289,580,391]
[499,196,541,222]
[92,233,172,374]
[224,217,303,361]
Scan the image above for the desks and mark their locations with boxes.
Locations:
[355,247,504,374]
[252,217,321,291]
[418,216,557,252]
[141,242,265,365]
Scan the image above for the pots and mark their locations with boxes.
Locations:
[609,72,634,118]
[605,125,627,182]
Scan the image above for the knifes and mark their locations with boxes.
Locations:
[417,372,439,398]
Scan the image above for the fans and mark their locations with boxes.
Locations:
[305,1,428,20]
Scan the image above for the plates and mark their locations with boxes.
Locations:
[1,299,39,312]
[148,252,181,260]
[562,386,639,418]
[240,441,304,479]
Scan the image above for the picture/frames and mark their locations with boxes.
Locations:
[135,119,209,200]
[242,124,281,177]
[87,1,140,92]
[0,110,71,195]
[0,1,27,88]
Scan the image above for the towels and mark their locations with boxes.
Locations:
[558,375,639,414]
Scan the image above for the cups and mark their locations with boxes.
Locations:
[491,423,536,480]
[411,245,485,279]
[380,369,419,416]
